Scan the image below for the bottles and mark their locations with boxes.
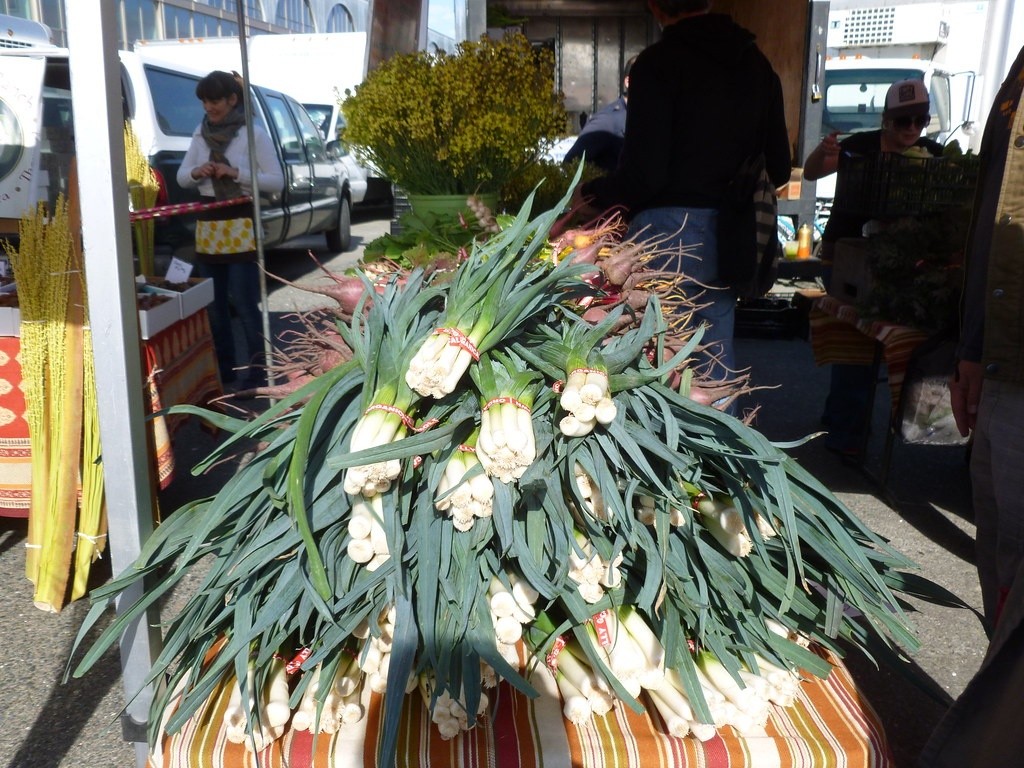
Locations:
[796,222,811,260]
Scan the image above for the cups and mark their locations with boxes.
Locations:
[786,240,799,261]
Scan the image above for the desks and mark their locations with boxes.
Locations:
[0,309,224,517]
[809,296,927,514]
[142,634,890,768]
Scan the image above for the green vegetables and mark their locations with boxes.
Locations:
[344,207,469,270]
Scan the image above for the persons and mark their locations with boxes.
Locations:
[563,0,792,419]
[803,78,982,438]
[178,71,286,400]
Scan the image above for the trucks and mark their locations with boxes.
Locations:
[454,0,824,297]
[810,1,985,241]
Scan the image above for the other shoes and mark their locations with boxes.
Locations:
[234,377,266,401]
[225,386,244,419]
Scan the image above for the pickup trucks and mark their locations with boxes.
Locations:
[298,97,391,208]
[0,15,353,284]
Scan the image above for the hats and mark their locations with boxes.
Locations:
[884,78,930,120]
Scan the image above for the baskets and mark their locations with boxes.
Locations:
[832,152,979,214]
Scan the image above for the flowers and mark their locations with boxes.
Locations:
[337,28,574,193]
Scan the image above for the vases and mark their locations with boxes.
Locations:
[406,193,496,229]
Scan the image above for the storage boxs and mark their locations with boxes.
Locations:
[0,282,213,340]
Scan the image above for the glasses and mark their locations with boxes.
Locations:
[559,206,602,229]
[887,112,931,131]
